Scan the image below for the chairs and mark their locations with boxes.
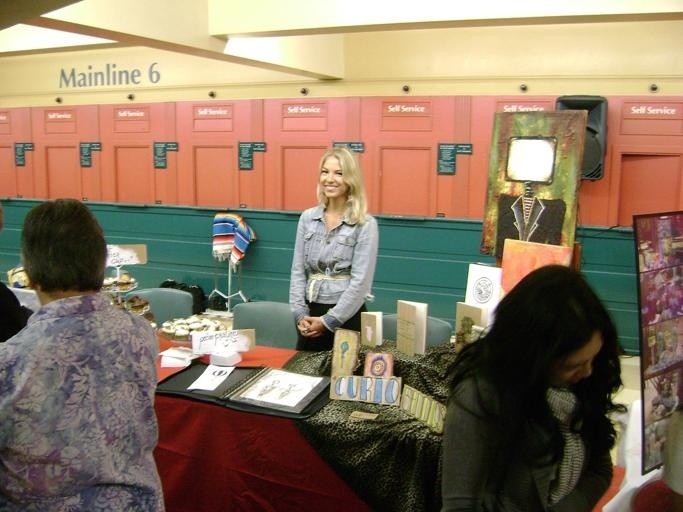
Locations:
[126,286,194,327]
[233,300,301,352]
[380,310,452,353]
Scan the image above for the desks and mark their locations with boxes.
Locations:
[154,327,463,512]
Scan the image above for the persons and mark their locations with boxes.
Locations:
[440,265,628,512]
[0,198,165,511]
[288,146,379,352]
[0,206,34,342]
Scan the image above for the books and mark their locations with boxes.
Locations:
[362,352,394,377]
[455,238,574,344]
[330,327,361,375]
[154,361,332,419]
[361,311,383,348]
[395,299,428,357]
